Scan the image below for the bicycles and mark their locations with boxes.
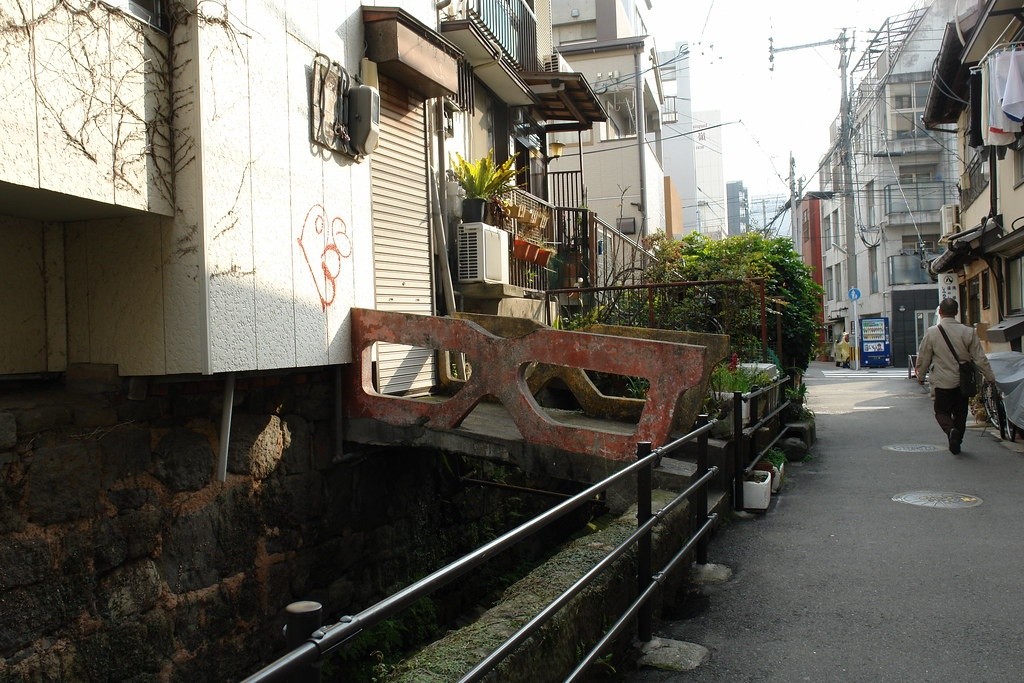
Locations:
[980,374,1017,442]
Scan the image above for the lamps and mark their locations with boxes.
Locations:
[547,140,566,164]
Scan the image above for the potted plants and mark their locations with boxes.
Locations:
[715,362,759,419]
[445,146,530,223]
[514,226,558,266]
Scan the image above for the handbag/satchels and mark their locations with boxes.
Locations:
[959,362,976,397]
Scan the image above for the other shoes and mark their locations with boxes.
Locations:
[949,428,960,455]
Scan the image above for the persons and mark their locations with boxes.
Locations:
[915,298,997,456]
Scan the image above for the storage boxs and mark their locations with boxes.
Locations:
[753,446,788,493]
[733,470,772,509]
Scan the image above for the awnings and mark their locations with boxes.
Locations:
[923,0,990,125]
[931,216,996,274]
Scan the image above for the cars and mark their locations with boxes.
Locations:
[833,331,855,368]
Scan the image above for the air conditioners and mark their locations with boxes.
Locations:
[542,53,574,72]
[457,222,509,284]
